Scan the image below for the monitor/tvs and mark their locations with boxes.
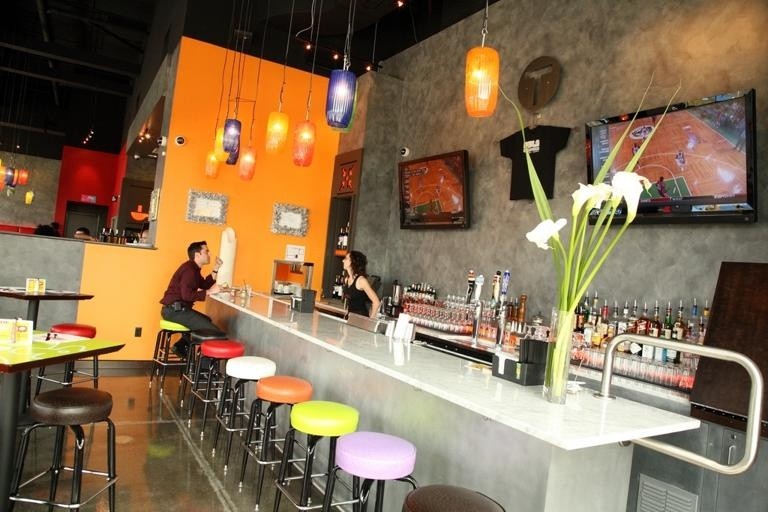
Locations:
[584,89,757,225]
[397,150,470,229]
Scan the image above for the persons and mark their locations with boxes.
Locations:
[75,227,89,235]
[631,98,746,199]
[341,250,380,321]
[159,241,223,359]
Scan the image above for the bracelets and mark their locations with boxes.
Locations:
[212,270,218,273]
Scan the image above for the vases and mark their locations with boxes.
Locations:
[541,309,575,404]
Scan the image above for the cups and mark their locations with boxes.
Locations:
[519,339,548,364]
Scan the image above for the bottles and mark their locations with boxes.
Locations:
[336,222,349,249]
[383,270,553,342]
[332,271,345,298]
[572,290,711,389]
[230,288,248,298]
[230,298,248,308]
[100,227,127,245]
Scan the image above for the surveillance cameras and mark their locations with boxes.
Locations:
[400,147,409,156]
[175,136,185,145]
[156,136,166,146]
[134,155,140,160]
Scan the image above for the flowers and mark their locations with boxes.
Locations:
[499,74,682,395]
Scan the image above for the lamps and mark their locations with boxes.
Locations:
[266,0,296,154]
[0,56,39,204]
[206,0,271,181]
[293,0,323,167]
[465,0,500,117]
[326,0,356,128]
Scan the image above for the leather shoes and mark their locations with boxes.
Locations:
[172,337,189,358]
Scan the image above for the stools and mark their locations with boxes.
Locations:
[212,356,276,471]
[9,387,116,512]
[401,486,504,512]
[324,431,419,512]
[149,320,190,393]
[181,329,227,413]
[188,340,245,436]
[35,324,98,397]
[273,400,359,512]
[238,375,313,511]
